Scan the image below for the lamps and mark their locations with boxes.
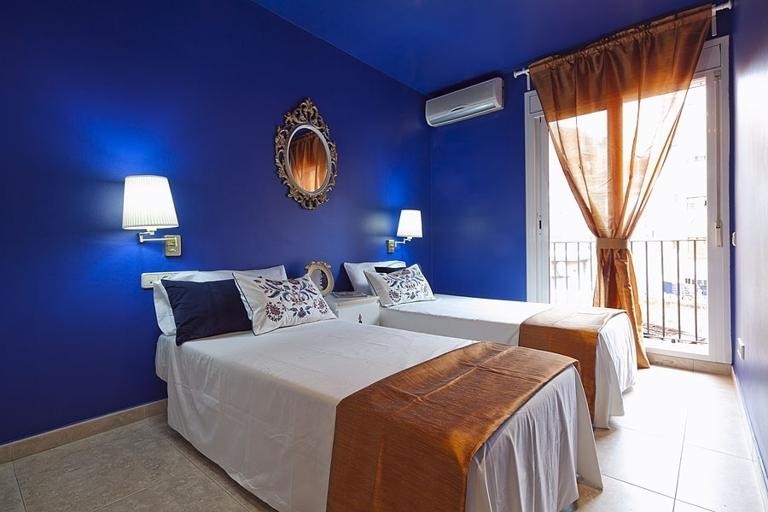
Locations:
[121,175,184,257]
[387,207,425,255]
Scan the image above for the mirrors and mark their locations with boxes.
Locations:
[273,97,338,211]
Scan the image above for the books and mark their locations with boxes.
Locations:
[332,291,368,301]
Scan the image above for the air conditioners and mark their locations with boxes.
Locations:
[423,75,505,129]
